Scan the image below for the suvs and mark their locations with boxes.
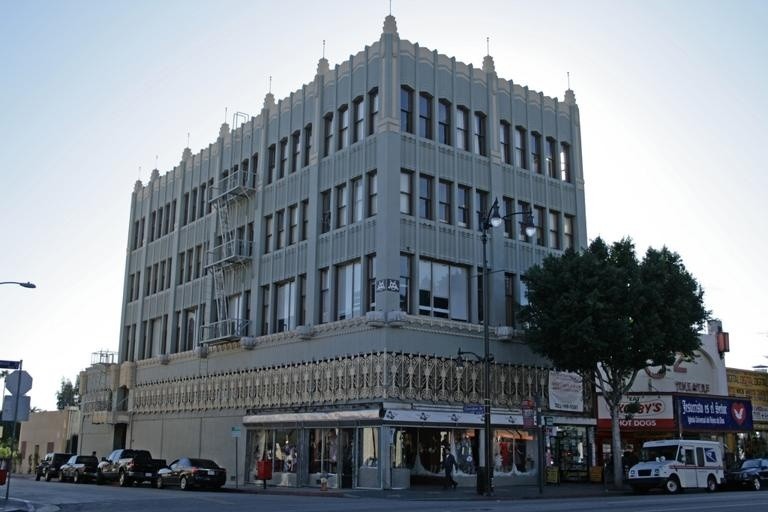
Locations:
[33,452,73,481]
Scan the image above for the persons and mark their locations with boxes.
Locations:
[622,444,638,469]
[441,448,458,490]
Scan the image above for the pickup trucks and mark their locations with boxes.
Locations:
[98,449,166,487]
[60,455,98,483]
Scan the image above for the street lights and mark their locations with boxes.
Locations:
[0,280,35,291]
[454,196,536,497]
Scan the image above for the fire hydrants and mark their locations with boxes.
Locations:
[320,473,332,491]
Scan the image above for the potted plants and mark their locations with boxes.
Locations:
[0,446,11,485]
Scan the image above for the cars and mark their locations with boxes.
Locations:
[725,457,767,492]
[156,457,227,490]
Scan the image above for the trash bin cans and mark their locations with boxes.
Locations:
[320,477,328,491]
[258,461,272,488]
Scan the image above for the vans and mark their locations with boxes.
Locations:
[626,439,723,495]
